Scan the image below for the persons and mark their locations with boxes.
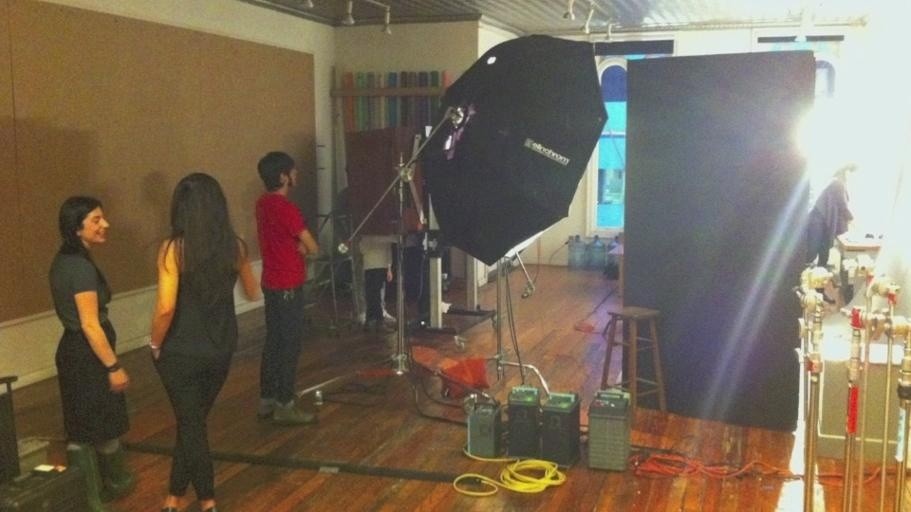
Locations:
[149,173,261,512]
[48,194,134,499]
[255,152,320,424]
[335,186,397,332]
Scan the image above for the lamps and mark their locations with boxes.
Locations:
[297,0,393,35]
[563,0,616,41]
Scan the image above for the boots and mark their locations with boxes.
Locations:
[96,446,136,504]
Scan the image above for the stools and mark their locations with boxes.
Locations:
[602,306,667,422]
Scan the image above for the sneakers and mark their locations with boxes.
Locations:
[161,507,178,512]
[256,396,275,420]
[364,318,395,332]
[203,504,220,512]
[273,399,318,426]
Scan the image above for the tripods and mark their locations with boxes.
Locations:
[296,107,492,406]
[459,255,553,398]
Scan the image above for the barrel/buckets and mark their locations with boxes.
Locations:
[607,238,620,265]
[584,234,607,270]
[568,234,588,271]
